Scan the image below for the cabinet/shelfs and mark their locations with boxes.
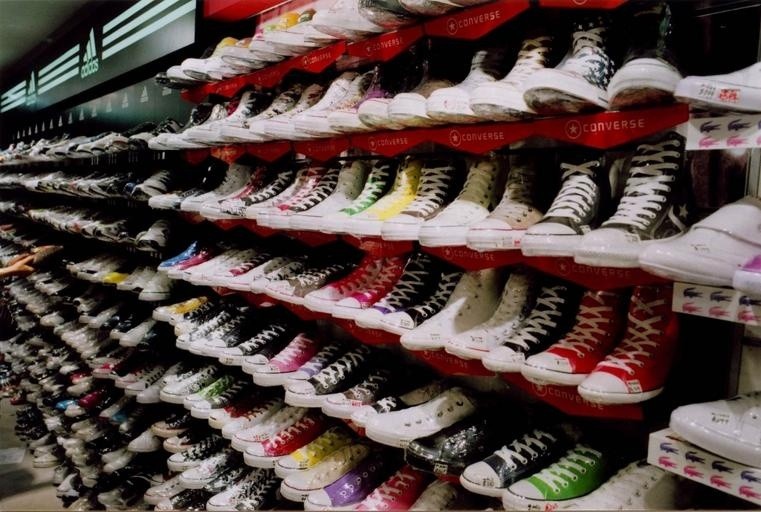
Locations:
[150,0,697,512]
[643,105,761,511]
[1,140,175,510]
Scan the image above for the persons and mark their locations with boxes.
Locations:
[0,253,35,276]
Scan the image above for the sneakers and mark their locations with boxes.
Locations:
[147,0,761,154]
[2,132,161,510]
[159,146,759,511]
